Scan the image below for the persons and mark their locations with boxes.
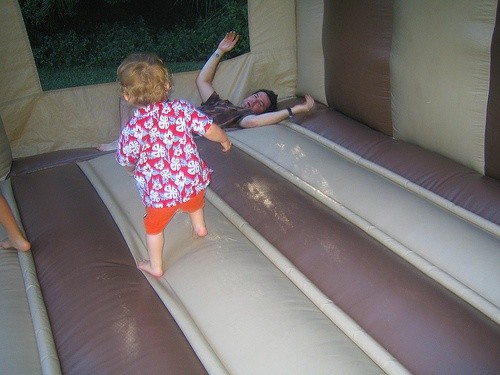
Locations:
[0,192,31,253]
[116,51,231,277]
[96,31,315,151]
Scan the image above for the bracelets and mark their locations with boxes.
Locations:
[214,53,221,59]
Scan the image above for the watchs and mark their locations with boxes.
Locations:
[286,107,293,118]
